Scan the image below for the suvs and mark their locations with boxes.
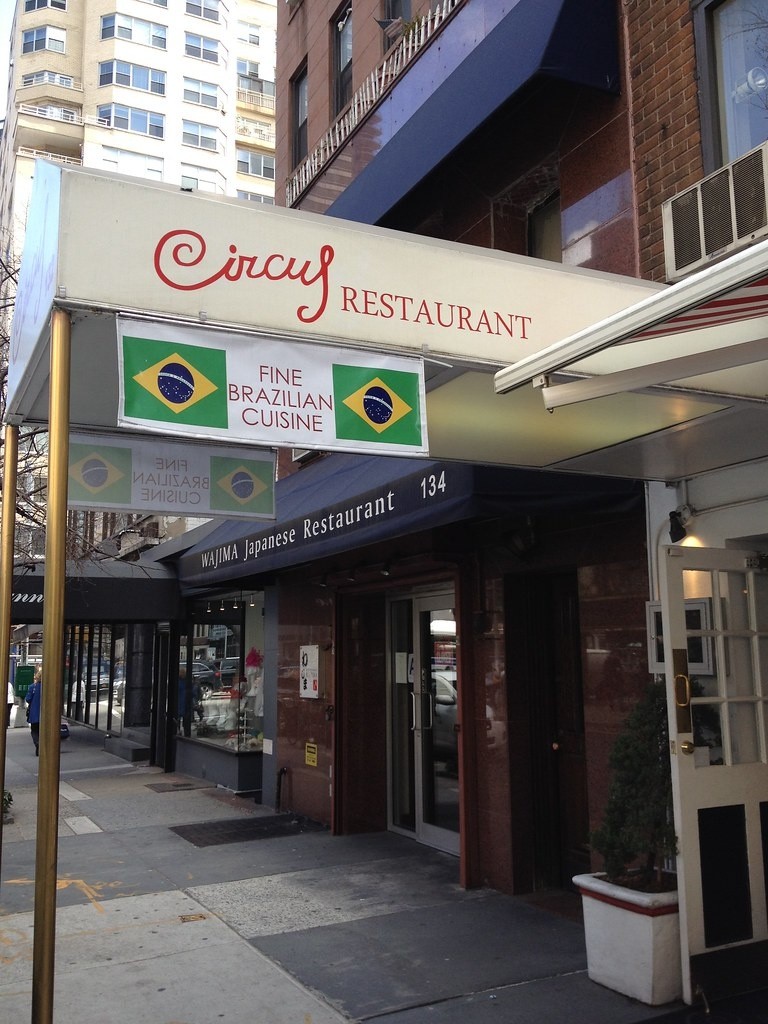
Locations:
[178,659,223,699]
[212,657,240,688]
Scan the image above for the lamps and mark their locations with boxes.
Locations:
[669,510,687,544]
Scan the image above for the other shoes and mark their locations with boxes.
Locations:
[191,719,194,722]
[35,743,39,757]
[200,715,204,721]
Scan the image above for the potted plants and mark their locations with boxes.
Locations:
[571,676,726,1003]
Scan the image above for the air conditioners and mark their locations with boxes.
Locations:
[661,140,768,283]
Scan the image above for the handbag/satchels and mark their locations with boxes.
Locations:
[193,700,204,712]
[26,704,31,722]
[60,724,70,739]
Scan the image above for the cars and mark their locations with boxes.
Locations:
[432,673,507,751]
[82,661,110,693]
[112,664,127,700]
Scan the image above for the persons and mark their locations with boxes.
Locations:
[26,671,42,756]
[177,668,196,735]
[8,682,14,726]
[71,676,85,721]
